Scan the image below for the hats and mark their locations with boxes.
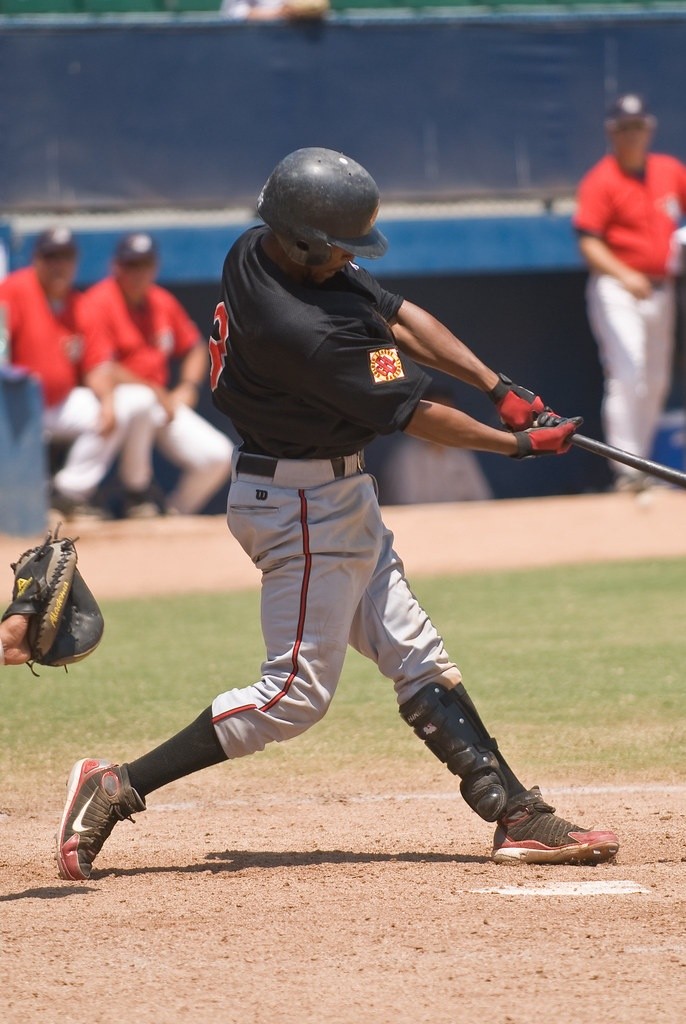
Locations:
[605,93,646,127]
[116,232,155,263]
[39,225,72,257]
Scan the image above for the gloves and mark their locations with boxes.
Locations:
[487,372,545,432]
[509,411,584,461]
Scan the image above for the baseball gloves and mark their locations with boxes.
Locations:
[9,522,105,679]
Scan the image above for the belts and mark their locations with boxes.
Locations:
[237,454,345,476]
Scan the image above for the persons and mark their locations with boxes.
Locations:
[81,230,237,519]
[55,145,620,879]
[0,566,60,675]
[570,92,686,494]
[382,385,490,505]
[2,222,117,523]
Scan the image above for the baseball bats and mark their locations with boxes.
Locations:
[531,419,686,486]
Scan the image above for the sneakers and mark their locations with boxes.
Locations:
[493,785,619,862]
[51,757,147,880]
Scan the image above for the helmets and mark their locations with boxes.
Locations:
[255,147,390,266]
